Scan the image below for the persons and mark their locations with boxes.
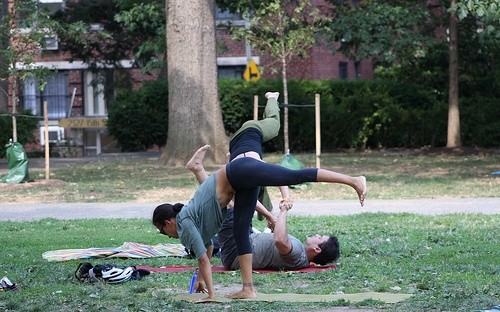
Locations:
[229,92,292,226]
[185,145,340,271]
[152,157,366,303]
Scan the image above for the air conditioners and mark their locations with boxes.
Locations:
[40,126,65,145]
